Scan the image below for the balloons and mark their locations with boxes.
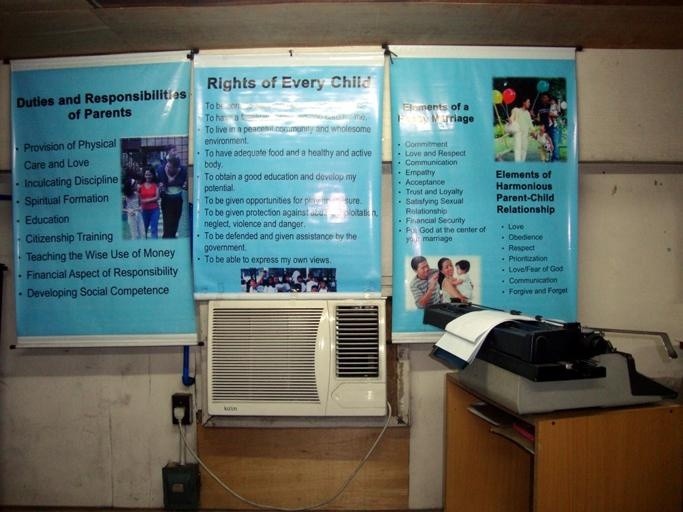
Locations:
[503,88,516,104]
[561,101,567,110]
[493,90,503,104]
[536,81,550,93]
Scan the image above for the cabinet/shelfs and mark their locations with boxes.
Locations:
[441,374,682,512]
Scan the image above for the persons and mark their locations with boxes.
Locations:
[438,258,467,304]
[409,256,442,309]
[240,270,335,293]
[506,93,561,162]
[122,148,186,238]
[447,260,474,304]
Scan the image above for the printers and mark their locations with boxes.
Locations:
[423,301,679,415]
